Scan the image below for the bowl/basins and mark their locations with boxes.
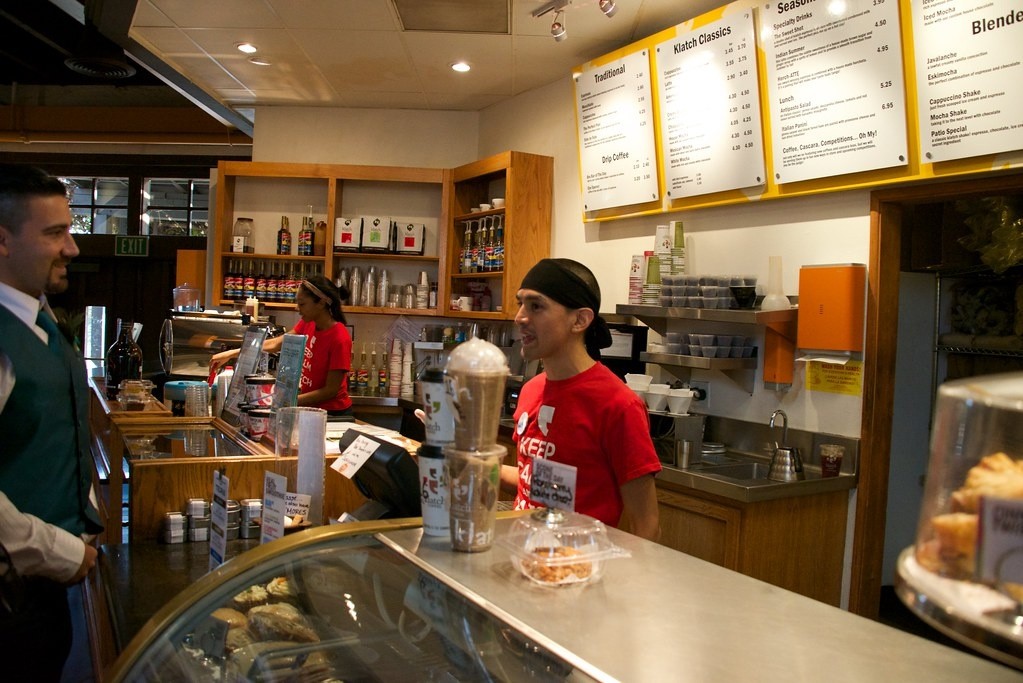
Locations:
[492,199,505,209]
[493,506,633,587]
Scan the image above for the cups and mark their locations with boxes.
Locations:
[457,296,472,312]
[675,439,693,469]
[470,321,511,347]
[653,221,686,276]
[416,339,513,553]
[390,338,414,399]
[624,374,693,416]
[660,274,766,310]
[470,204,491,212]
[337,266,429,309]
[237,373,327,456]
[666,332,755,358]
[629,251,662,306]
[819,444,845,478]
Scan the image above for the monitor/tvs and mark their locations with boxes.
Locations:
[339,429,422,521]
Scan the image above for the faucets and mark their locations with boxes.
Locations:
[769,409,788,449]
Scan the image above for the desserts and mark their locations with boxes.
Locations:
[195,574,340,683]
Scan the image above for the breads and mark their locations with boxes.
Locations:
[522,544,592,583]
[924,450,1023,610]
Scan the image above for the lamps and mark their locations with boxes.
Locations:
[551,0,572,43]
[599,0,620,18]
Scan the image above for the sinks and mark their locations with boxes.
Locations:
[695,462,771,481]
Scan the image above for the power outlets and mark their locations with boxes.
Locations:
[691,381,710,409]
[424,352,435,370]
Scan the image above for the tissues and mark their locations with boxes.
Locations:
[794,262,868,367]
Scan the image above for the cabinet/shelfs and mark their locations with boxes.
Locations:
[650,479,849,607]
[615,295,800,397]
[211,160,451,316]
[443,151,554,318]
[496,435,520,502]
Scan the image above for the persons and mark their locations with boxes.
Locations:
[413,259,662,544]
[209,275,353,416]
[0,160,105,683]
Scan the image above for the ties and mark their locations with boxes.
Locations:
[35,311,61,345]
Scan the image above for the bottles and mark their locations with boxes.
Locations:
[454,322,471,344]
[224,258,321,326]
[481,290,491,311]
[215,366,235,417]
[230,216,327,256]
[105,320,142,402]
[429,282,438,309]
